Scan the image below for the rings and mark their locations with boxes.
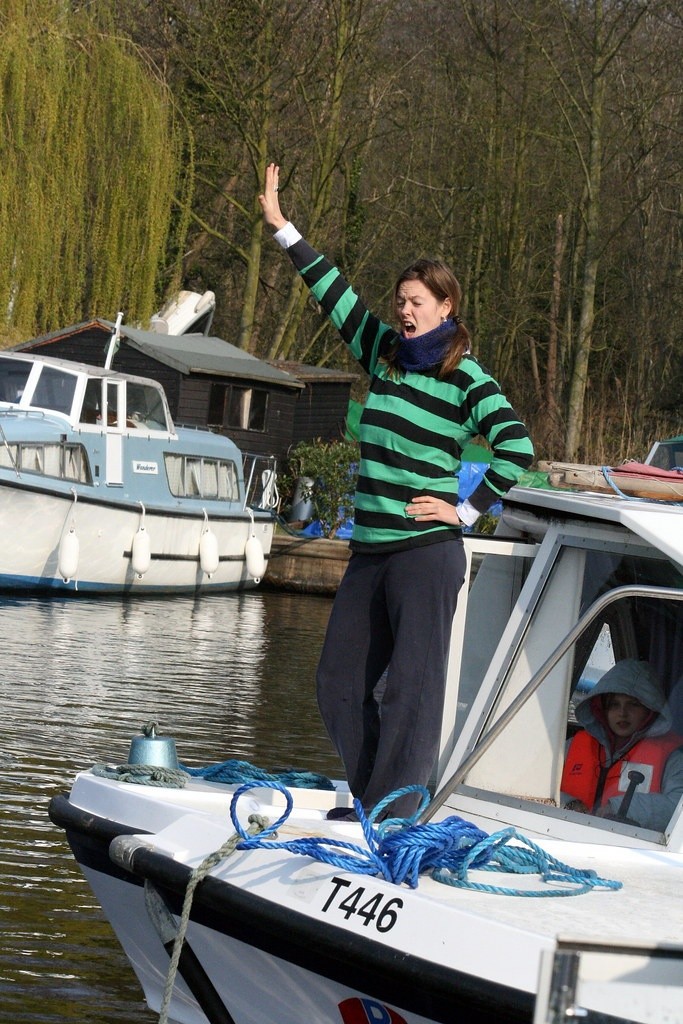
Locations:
[273,187,279,192]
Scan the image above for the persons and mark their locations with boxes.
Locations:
[558,658,683,834]
[260,161,535,837]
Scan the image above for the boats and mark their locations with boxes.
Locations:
[0,311,278,597]
[48,442,683,1022]
